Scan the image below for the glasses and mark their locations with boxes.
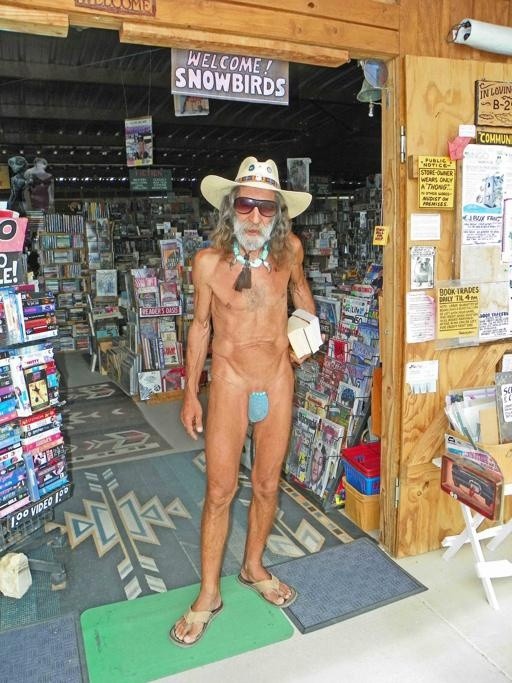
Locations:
[234,198,279,217]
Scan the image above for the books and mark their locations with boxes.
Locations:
[125,114,154,167]
[0,181,382,520]
[173,94,210,117]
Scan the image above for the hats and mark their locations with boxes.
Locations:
[200,156,312,219]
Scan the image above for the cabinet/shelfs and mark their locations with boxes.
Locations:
[69,195,223,401]
[0,201,75,542]
[287,179,382,508]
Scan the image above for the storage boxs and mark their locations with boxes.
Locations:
[447,421,511,483]
[340,443,383,532]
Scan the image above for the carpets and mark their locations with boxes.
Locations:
[266,541,429,633]
[80,574,294,682]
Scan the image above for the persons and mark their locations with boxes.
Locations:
[28,157,55,212]
[168,155,328,648]
[134,141,149,160]
[6,154,29,218]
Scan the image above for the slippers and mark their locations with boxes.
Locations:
[237,572,298,608]
[169,601,225,647]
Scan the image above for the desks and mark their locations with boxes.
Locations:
[432,456,511,607]
